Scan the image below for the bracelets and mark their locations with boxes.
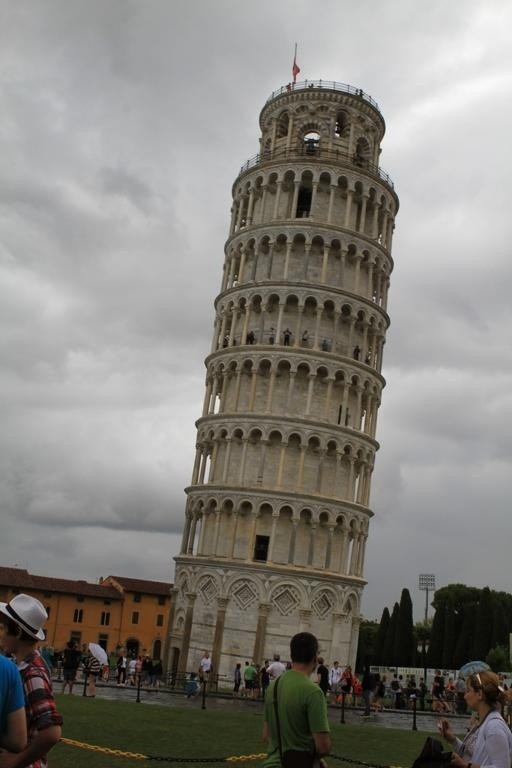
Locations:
[467,761,473,768]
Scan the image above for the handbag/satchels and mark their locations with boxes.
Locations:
[283,750,313,768]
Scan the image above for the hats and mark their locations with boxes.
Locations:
[0,594,48,641]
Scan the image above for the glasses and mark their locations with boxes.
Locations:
[473,672,481,684]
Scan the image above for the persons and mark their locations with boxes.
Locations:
[314,657,469,716]
[185,652,291,701]
[223,326,388,366]
[257,633,333,766]
[37,641,165,698]
[437,669,512,768]
[0,590,64,768]
[0,654,29,755]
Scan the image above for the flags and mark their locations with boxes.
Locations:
[293,61,301,76]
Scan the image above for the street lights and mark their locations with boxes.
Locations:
[418,573,437,682]
